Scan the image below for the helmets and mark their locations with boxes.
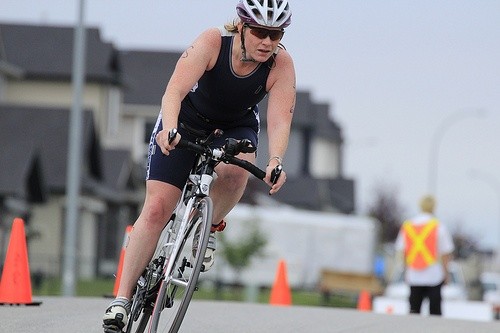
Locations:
[236,0,292,30]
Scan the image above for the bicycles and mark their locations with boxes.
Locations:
[118,128,282,333]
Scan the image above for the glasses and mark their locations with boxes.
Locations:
[247,24,284,41]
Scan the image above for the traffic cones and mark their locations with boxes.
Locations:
[103,224,133,298]
[0,218,44,307]
[357,289,373,312]
[269,259,294,306]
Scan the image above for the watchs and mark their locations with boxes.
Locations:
[267,155,284,165]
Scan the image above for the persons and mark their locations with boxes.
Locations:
[102,1,296,331]
[394,196,455,316]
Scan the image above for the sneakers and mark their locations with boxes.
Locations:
[103,298,131,329]
[190,221,217,272]
